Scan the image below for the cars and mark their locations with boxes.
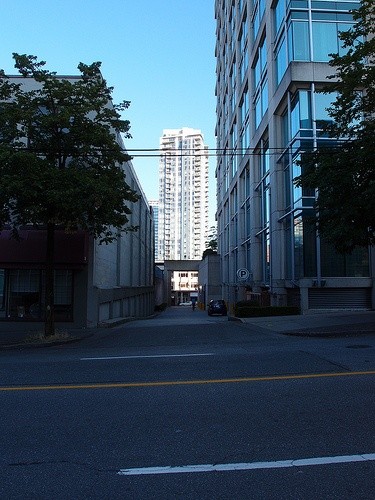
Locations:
[207,300,228,316]
[179,301,192,306]
